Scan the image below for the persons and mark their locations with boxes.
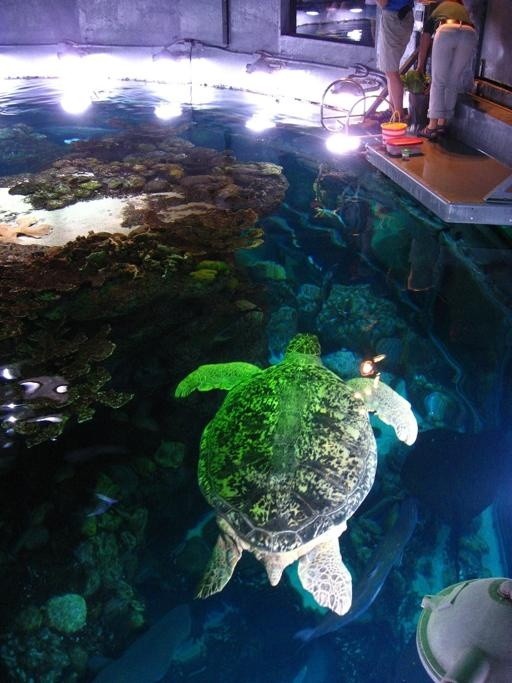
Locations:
[375,0,418,124]
[416,0,478,139]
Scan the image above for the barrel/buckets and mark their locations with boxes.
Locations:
[380,110,408,150]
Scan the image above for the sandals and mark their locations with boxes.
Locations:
[418,125,443,137]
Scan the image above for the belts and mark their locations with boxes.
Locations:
[435,19,471,28]
